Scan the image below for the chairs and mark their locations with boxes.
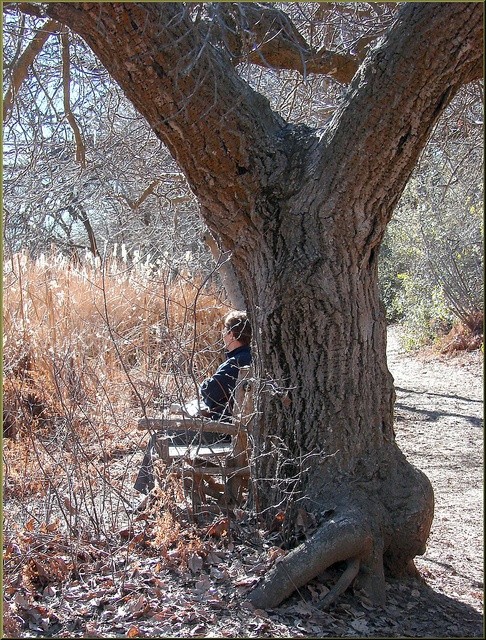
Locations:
[137,366,254,507]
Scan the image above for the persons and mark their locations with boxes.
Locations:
[127,311,252,516]
